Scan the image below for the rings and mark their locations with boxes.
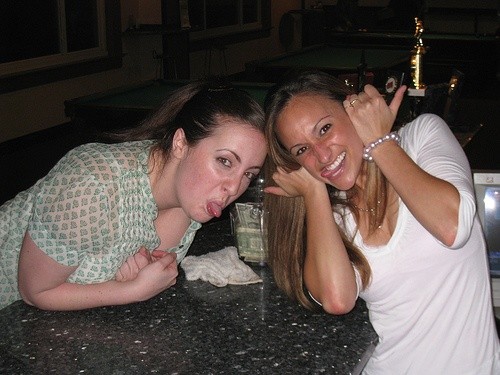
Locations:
[350,96,361,106]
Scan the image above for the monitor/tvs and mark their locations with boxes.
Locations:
[472,173,499,307]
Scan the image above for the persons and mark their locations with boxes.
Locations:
[257,66,500,375]
[0,78,273,310]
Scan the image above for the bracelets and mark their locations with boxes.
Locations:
[363,133,402,164]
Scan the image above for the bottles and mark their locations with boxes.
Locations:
[250,173,270,266]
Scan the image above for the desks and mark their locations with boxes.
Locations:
[0,177,379,375]
[63,77,278,131]
[243,39,429,87]
